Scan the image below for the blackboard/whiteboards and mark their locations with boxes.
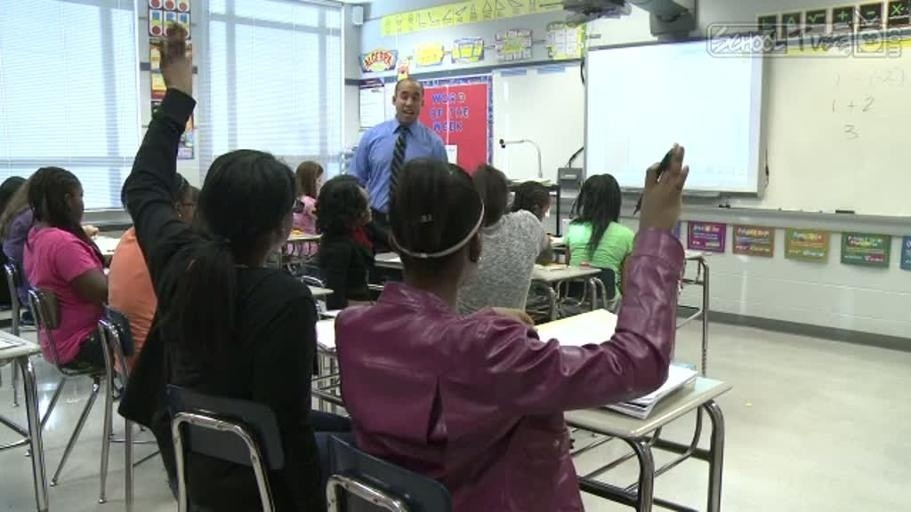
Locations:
[491,27,911,225]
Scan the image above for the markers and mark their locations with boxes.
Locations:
[717,203,731,209]
[836,210,855,214]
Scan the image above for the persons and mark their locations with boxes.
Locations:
[1,162,636,376]
[347,77,449,254]
[127,29,353,512]
[336,142,690,512]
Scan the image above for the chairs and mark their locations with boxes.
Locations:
[323,431,453,512]
[166,383,287,512]
[4,263,38,410]
[298,275,349,418]
[98,305,164,512]
[25,288,147,487]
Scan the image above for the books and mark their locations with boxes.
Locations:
[533,308,701,421]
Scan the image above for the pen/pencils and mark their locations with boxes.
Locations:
[631,148,673,216]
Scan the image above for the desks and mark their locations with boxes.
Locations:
[311,310,733,510]
[92,228,708,379]
[0,330,48,511]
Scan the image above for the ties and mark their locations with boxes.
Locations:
[389,128,408,207]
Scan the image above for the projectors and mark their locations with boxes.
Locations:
[562,0,632,15]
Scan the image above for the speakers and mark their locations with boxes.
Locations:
[352,5,363,24]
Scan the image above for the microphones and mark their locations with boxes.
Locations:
[500,138,549,183]
